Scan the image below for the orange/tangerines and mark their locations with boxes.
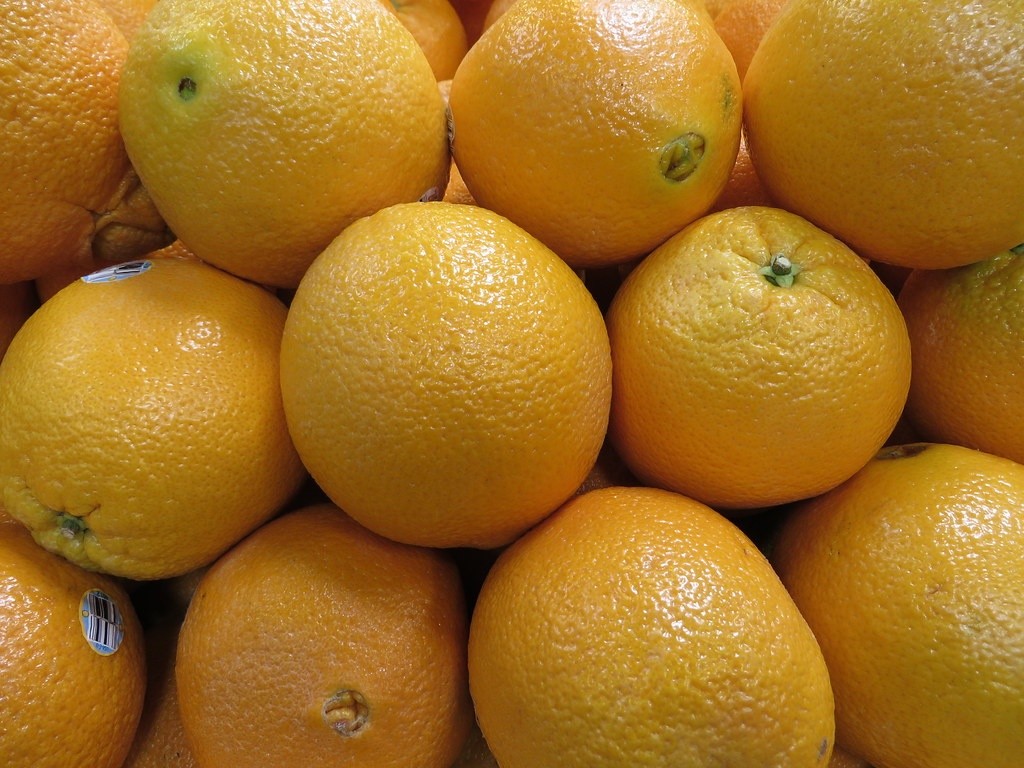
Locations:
[0,0,1024,768]
